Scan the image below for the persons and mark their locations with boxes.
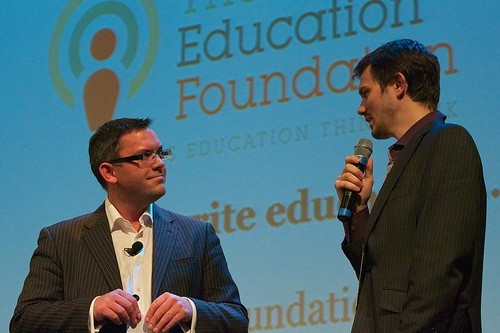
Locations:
[6,118,250,333]
[334,38,487,332]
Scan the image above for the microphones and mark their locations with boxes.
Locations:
[124,241,143,256]
[337,139,373,223]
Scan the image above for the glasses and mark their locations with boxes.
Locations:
[109,148,172,164]
